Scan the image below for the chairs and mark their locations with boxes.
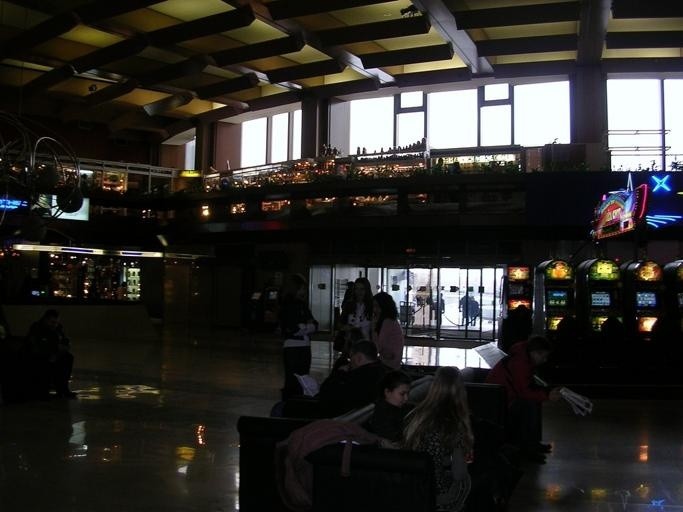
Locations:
[236,364,542,512]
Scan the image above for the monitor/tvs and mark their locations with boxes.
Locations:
[545,288,569,306]
[635,291,658,308]
[505,282,531,299]
[591,291,611,306]
[49,194,90,223]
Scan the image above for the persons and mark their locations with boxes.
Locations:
[375,364,477,510]
[366,370,426,469]
[341,280,355,309]
[24,308,77,404]
[429,292,479,327]
[371,293,406,371]
[332,276,375,359]
[407,306,417,325]
[268,339,398,421]
[276,274,319,395]
[486,333,563,464]
